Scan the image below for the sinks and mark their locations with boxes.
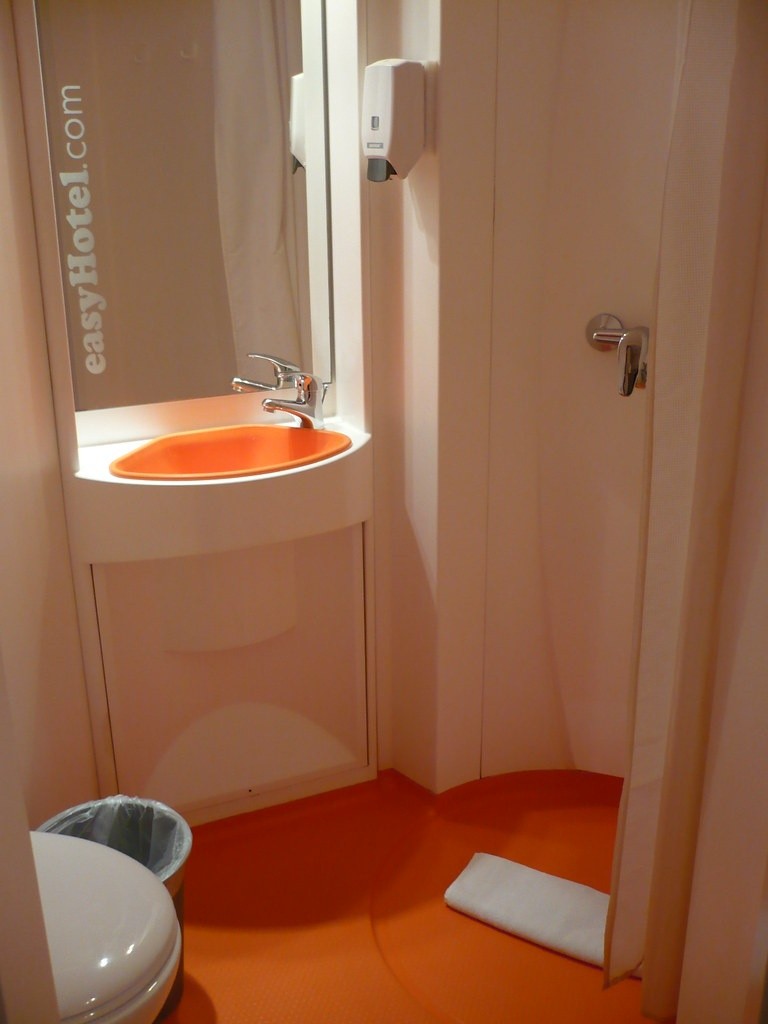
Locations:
[110,421,352,480]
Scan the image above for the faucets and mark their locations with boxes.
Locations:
[261,370,329,432]
[229,351,300,393]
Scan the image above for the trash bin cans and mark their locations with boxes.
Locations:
[33,794,195,1015]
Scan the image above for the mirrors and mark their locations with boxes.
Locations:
[34,0,334,412]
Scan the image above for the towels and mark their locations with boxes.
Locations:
[444,850,613,968]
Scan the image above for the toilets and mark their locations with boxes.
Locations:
[25,827,181,1024]
